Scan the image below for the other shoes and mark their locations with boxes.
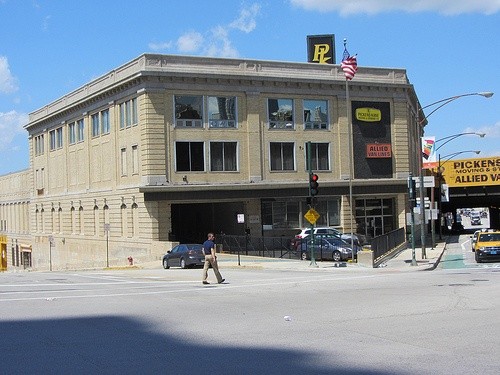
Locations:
[218,279,225,284]
[203,281,210,284]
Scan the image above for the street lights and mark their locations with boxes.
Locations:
[416,92,495,259]
[438,150,481,240]
[429,132,487,248]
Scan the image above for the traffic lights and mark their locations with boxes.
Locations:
[309,172,319,196]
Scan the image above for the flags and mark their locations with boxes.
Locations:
[341,48,358,81]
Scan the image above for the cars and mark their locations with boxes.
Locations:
[459,209,500,263]
[289,227,359,261]
[162,244,216,269]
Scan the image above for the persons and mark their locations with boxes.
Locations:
[202,233,226,285]
